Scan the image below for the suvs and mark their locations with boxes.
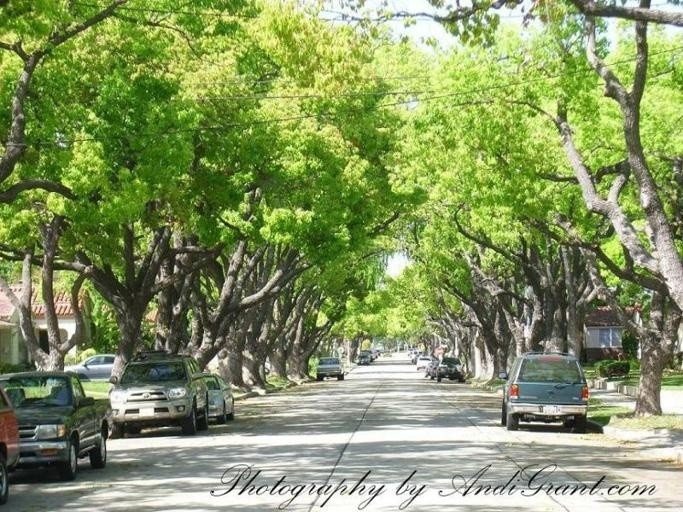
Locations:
[108,350,209,436]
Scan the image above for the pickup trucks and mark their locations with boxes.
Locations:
[0,370,110,480]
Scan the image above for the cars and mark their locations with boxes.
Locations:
[315,357,344,380]
[500,350,588,434]
[357,348,380,365]
[0,386,20,505]
[64,354,115,378]
[193,373,235,423]
[411,349,464,384]
